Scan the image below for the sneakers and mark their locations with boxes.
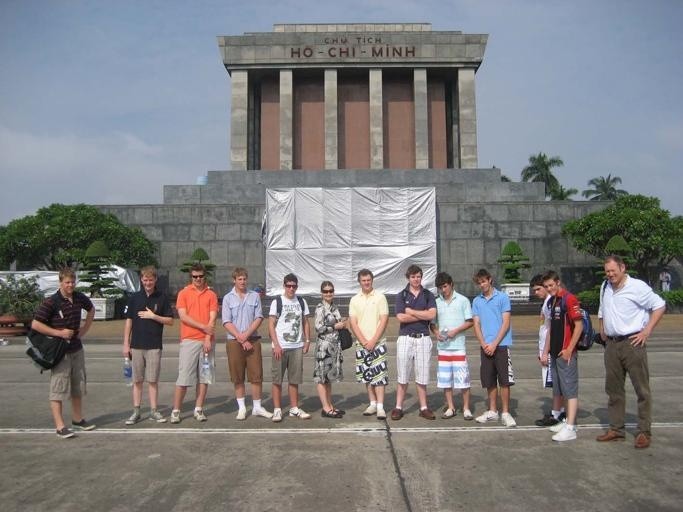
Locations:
[236,408,247,420]
[72,419,95,431]
[363,406,387,420]
[272,408,282,421]
[149,411,167,422]
[56,427,74,438]
[290,408,311,419]
[442,409,516,427]
[252,406,272,418]
[194,410,208,421]
[535,412,578,441]
[170,410,181,423]
[125,412,141,424]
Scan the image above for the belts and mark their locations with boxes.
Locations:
[409,334,428,338]
[607,332,640,341]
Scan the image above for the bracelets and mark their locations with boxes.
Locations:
[306,339,311,343]
[640,328,650,337]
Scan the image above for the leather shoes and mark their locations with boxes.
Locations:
[322,407,345,418]
[635,433,649,448]
[597,430,625,442]
[419,409,435,420]
[392,408,403,420]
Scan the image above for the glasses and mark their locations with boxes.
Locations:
[285,285,297,288]
[193,275,203,278]
[323,289,334,293]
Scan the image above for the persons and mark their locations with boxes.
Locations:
[170,265,220,422]
[529,270,584,442]
[525,275,567,427]
[349,269,390,419]
[472,269,517,426]
[313,280,345,418]
[598,254,667,448]
[221,267,274,420]
[268,274,311,422]
[428,272,474,419]
[123,264,174,425]
[391,264,437,420]
[659,267,672,293]
[31,269,96,438]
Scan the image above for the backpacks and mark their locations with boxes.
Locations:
[562,293,595,350]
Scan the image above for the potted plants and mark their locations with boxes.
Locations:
[496,239,531,302]
[0,273,44,338]
[74,240,119,320]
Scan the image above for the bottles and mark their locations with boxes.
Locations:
[437,328,448,347]
[123,357,133,387]
[200,353,210,380]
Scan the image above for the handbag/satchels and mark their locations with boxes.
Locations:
[26,328,70,374]
[340,328,352,350]
